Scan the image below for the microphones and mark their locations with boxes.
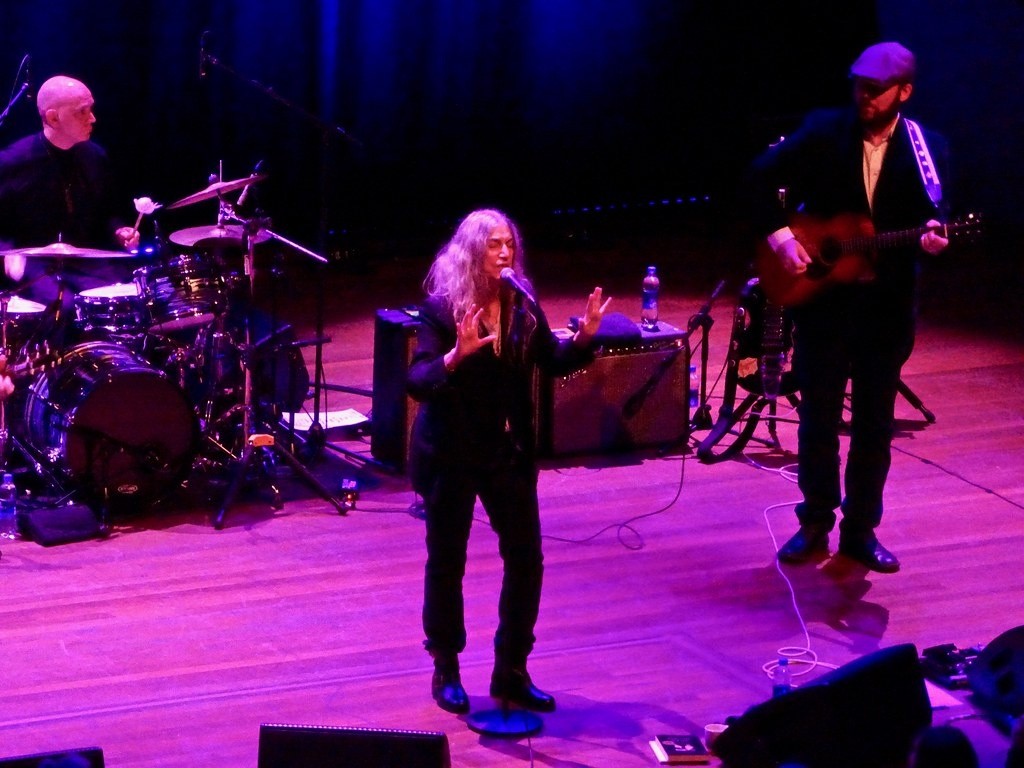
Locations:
[26,57,35,105]
[500,268,537,304]
[621,390,647,419]
[198,37,207,80]
[236,164,262,206]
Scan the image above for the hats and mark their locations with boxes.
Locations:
[848,41,915,87]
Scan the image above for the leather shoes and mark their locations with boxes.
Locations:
[839,517,900,571]
[778,523,829,562]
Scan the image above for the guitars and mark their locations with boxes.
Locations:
[736,135,799,395]
[757,211,985,307]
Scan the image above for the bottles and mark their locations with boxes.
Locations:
[0,473,16,539]
[641,266,660,329]
[689,365,700,423]
[773,657,791,701]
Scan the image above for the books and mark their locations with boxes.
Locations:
[655,735,708,761]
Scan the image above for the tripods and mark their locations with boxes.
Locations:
[0,258,74,513]
[642,280,779,457]
[176,203,395,529]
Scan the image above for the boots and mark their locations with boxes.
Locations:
[428,647,470,712]
[490,647,556,710]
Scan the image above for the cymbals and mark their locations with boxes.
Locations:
[167,173,265,209]
[169,224,273,247]
[0,243,135,257]
[1,296,47,314]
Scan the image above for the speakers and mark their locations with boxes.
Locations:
[257,723,451,768]
[370,307,423,475]
[531,319,690,460]
[0,747,105,768]
[967,625,1024,720]
[710,644,932,768]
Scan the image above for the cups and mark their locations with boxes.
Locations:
[705,723,729,748]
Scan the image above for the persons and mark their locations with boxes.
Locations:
[410,210,614,712]
[0,74,141,310]
[739,41,951,574]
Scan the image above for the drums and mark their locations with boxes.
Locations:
[74,280,153,353]
[132,250,232,332]
[24,339,198,503]
